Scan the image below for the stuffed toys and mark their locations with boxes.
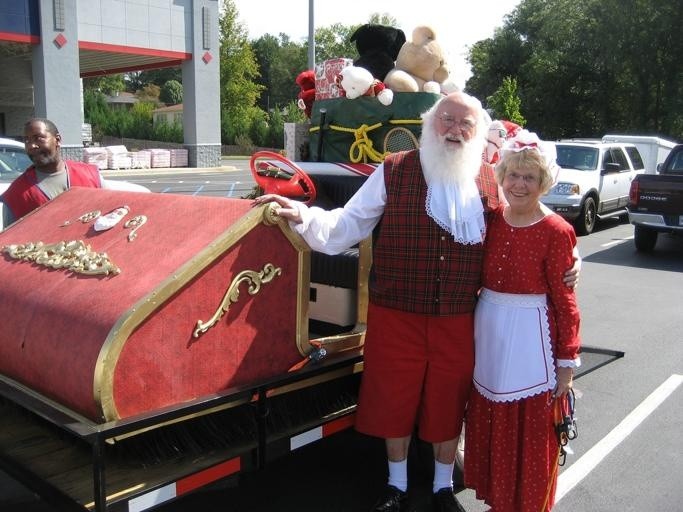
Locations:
[485,119,524,164]
[295,24,451,120]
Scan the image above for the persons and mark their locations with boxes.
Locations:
[0,118,107,230]
[462,129,580,512]
[253,92,580,512]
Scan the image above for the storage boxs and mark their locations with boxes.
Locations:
[312,58,356,98]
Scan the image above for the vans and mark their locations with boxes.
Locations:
[538,140,644,234]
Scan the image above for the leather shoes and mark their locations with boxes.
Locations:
[429,486,466,511]
[369,482,412,512]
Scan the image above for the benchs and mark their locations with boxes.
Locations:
[308,245,359,335]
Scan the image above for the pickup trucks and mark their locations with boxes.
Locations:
[626,144,681,256]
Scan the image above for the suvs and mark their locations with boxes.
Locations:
[0,136,152,236]
